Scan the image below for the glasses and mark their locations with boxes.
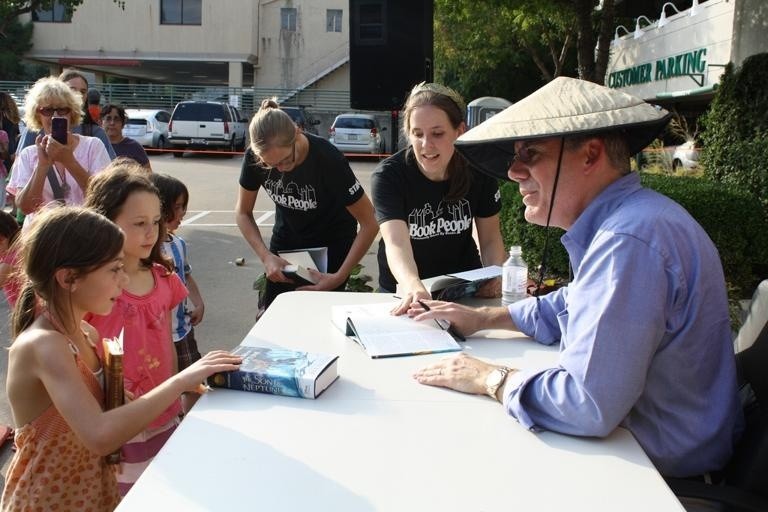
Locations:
[38,106,72,116]
[262,143,295,170]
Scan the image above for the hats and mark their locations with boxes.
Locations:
[451,75,674,185]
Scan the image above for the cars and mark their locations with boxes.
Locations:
[121,107,171,155]
[272,106,320,134]
[670,140,702,173]
[327,113,387,161]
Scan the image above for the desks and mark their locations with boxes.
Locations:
[113,291,688,512]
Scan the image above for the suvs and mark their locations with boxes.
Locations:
[167,99,248,160]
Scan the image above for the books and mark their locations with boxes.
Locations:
[103,336,124,466]
[205,334,340,400]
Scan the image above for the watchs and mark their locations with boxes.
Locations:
[486,365,514,402]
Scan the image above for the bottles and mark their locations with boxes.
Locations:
[499,243,529,310]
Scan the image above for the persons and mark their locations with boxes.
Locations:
[1,93,21,256]
[98,103,151,168]
[234,97,380,322]
[84,159,190,504]
[87,88,103,123]
[149,173,204,414]
[5,75,112,240]
[0,200,244,511]
[369,80,505,317]
[405,75,741,491]
[15,72,117,161]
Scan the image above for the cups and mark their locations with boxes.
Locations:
[235,257,244,265]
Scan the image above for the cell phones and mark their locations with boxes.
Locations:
[51,117,67,143]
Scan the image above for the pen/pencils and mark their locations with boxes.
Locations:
[417,300,466,341]
